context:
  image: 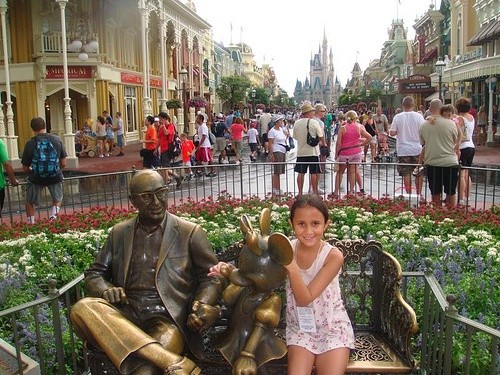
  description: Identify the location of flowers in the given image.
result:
[186,95,208,108]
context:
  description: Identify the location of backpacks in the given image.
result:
[31,133,61,179]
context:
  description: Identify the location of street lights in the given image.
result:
[435,57,446,103]
[179,65,189,134]
[383,81,390,119]
[251,89,256,114]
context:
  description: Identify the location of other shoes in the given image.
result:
[105,153,110,157]
[175,175,184,190]
[116,152,123,156]
[222,160,230,164]
[328,192,339,198]
[184,171,216,182]
[236,161,241,165]
[360,189,367,195]
[167,177,172,184]
[250,155,256,163]
[344,194,356,199]
[98,154,104,157]
[413,166,425,176]
[314,189,326,195]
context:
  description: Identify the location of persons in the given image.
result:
[328,110,372,200]
[73,170,228,375]
[413,98,476,209]
[390,96,427,205]
[468,104,497,146]
[293,102,331,196]
[75,111,125,158]
[137,106,246,187]
[0,139,19,226]
[247,100,394,172]
[268,114,290,196]
[20,118,67,225]
[210,195,357,375]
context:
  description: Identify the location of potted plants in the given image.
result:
[166,99,182,109]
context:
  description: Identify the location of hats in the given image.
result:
[301,104,317,113]
[272,114,283,123]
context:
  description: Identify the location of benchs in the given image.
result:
[82,237,420,375]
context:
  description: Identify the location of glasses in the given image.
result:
[321,110,327,113]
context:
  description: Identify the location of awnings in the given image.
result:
[441,54,500,82]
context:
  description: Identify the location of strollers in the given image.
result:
[218,136,242,164]
[377,133,393,163]
[75,133,97,157]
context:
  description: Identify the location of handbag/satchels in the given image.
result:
[458,116,471,142]
[286,145,291,151]
[289,137,295,149]
[209,132,217,144]
[307,132,319,147]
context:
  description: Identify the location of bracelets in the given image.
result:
[269,155,274,159]
